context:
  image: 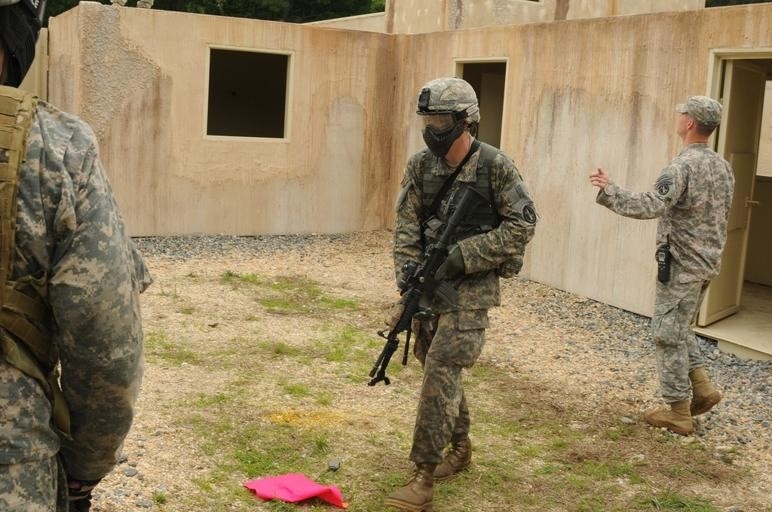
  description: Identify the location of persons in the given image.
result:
[381,75,544,511]
[1,0,156,512]
[588,95,736,436]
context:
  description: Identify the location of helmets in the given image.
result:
[417,77,478,111]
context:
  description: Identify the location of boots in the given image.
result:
[645,399,694,437]
[688,366,723,417]
[383,463,438,512]
[432,430,472,481]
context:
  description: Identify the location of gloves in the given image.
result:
[57,476,105,512]
[432,244,465,282]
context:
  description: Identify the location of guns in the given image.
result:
[367,186,489,386]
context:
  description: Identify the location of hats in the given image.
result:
[675,95,725,127]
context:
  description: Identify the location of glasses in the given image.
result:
[416,102,480,134]
